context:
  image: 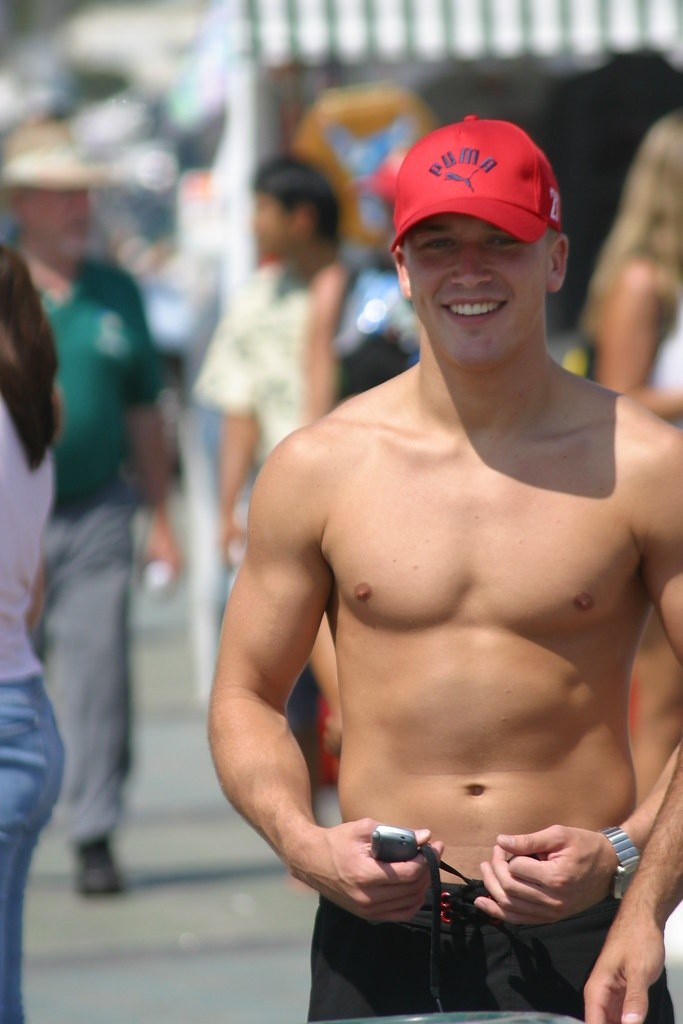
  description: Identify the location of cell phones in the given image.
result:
[369,825,418,864]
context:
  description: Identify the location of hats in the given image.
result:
[0,118,112,193]
[390,114,561,252]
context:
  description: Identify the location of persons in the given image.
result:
[210,117,683,1024]
[2,119,186,896]
[1,39,683,831]
[583,744,683,1024]
[1,245,66,1023]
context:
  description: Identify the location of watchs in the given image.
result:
[599,826,641,900]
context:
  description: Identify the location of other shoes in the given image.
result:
[73,842,121,895]
